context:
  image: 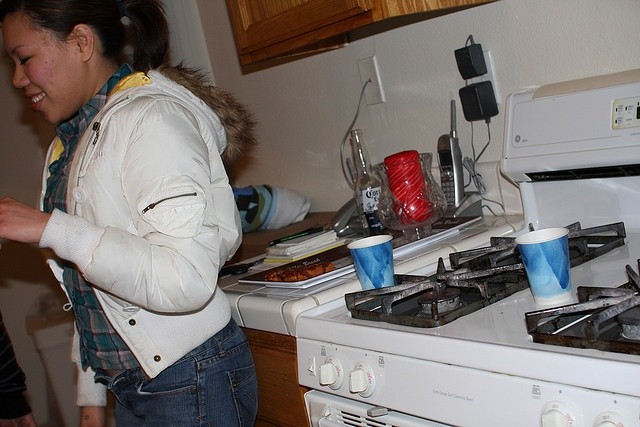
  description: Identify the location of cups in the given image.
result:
[346,235,395,291]
[371,149,449,243]
[515,229,572,307]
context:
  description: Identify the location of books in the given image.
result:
[248,226,347,272]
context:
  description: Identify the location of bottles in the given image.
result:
[350,128,387,237]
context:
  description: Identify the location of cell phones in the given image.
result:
[437,100,465,209]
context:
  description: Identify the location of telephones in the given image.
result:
[437,99,483,218]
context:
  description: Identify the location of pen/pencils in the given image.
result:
[268,227,323,246]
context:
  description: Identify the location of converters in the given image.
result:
[454,34,488,80]
[458,81,499,122]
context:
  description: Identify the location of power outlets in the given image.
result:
[466,49,501,104]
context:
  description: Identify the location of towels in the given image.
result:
[231,184,310,232]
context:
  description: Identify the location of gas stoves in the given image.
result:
[296,220,640,427]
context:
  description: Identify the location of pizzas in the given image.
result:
[263,260,336,282]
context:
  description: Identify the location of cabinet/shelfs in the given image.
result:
[225,0,500,76]
[239,325,310,427]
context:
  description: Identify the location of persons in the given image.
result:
[0,308,37,427]
[0,0,260,427]
